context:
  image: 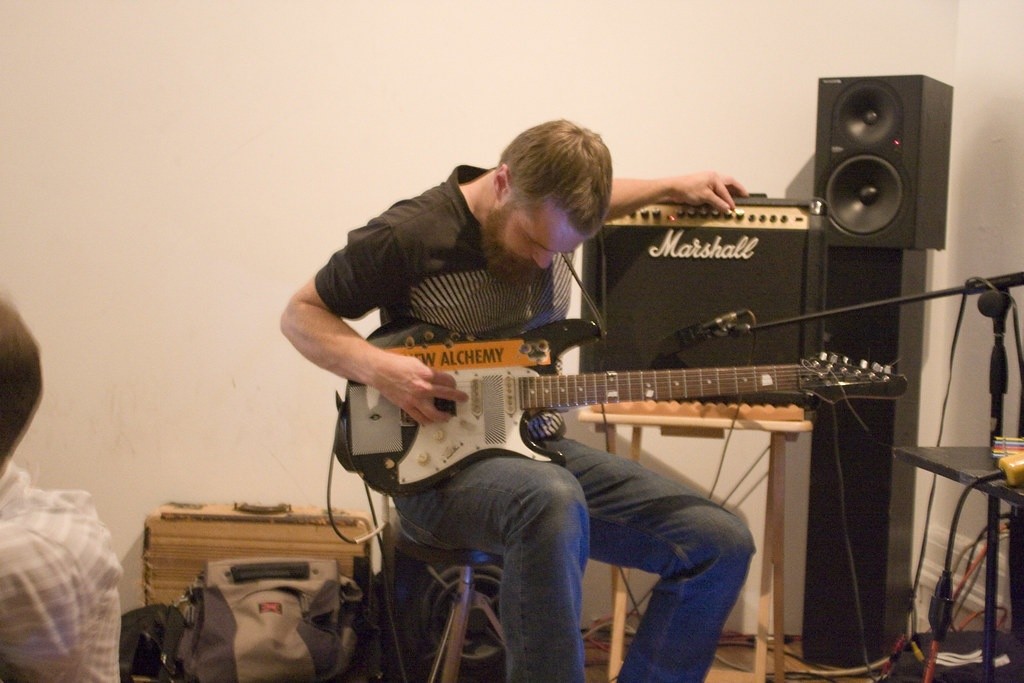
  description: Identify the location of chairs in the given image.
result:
[378,492,503,683]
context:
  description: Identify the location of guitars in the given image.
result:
[341,318,911,496]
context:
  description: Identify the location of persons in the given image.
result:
[279,121,755,683]
[0,303,122,683]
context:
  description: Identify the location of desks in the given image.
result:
[894,446,1024,683]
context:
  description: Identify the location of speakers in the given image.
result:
[814,74,955,252]
[390,534,509,683]
[579,196,828,413]
[802,248,927,672]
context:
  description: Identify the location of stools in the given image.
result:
[578,403,813,683]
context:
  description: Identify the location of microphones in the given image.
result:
[675,310,751,348]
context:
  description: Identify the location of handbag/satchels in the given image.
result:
[118,603,187,683]
[176,557,372,683]
